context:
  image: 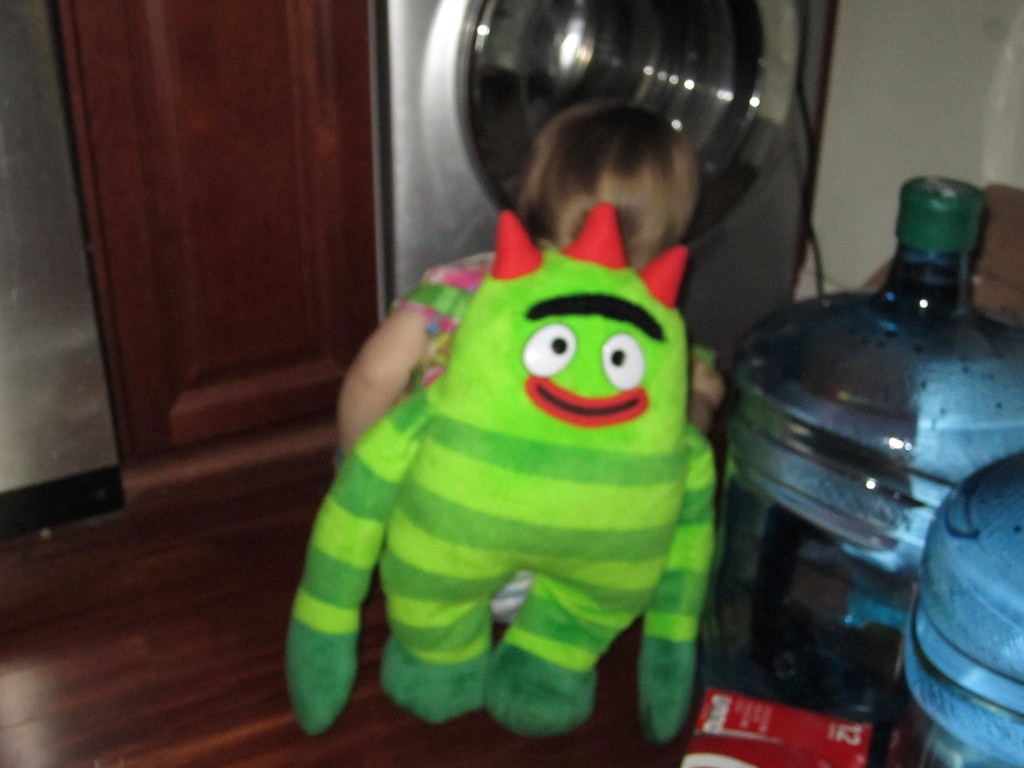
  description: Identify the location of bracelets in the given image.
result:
[336,453,343,466]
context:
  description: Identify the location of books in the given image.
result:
[679,687,871,768]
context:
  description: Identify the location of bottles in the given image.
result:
[870,450,1023,764]
[693,170,1024,727]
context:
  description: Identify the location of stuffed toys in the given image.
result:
[285,202,716,745]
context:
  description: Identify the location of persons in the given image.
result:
[332,101,726,621]
